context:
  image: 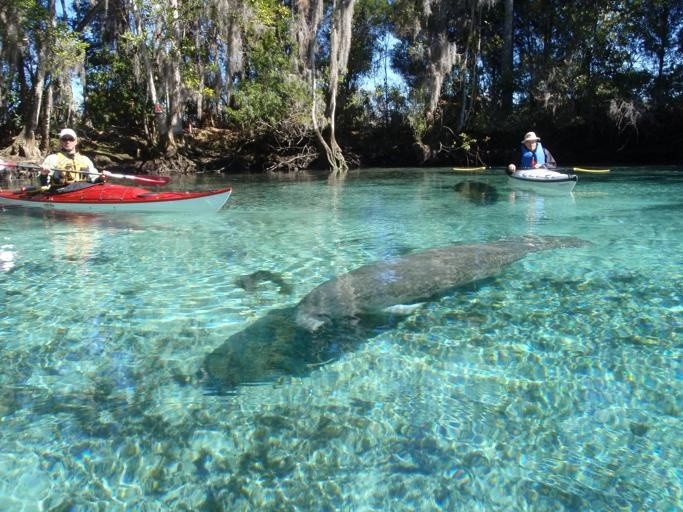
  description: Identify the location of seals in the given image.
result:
[294,236,596,351]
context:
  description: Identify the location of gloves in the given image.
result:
[508,163,515,172]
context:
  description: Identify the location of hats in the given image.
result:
[59,128,76,139]
[521,131,540,142]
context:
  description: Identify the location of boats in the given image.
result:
[511,169,578,181]
[0,181,233,213]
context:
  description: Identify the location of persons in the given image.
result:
[507,131,557,174]
[38,127,111,195]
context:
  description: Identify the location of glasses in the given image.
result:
[61,137,74,141]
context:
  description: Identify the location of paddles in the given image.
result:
[453,167,610,173]
[0,160,169,186]
